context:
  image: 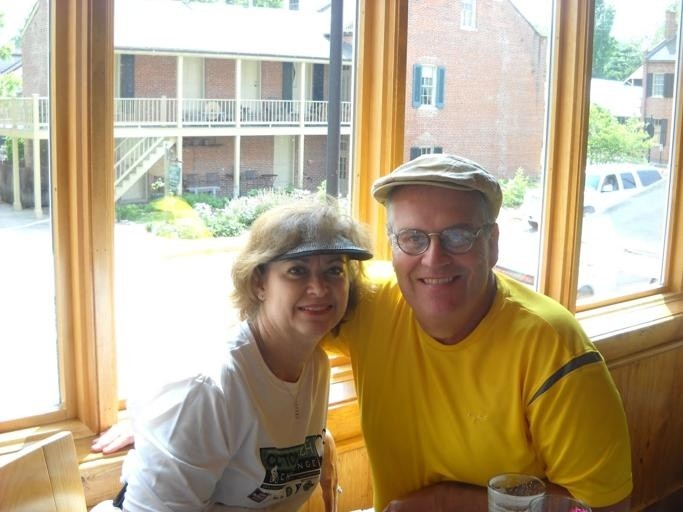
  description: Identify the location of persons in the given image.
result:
[88,198,376,512]
[88,153,637,512]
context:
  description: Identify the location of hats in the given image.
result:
[370,155,502,218]
[278,212,372,261]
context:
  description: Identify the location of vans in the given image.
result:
[522,164,664,230]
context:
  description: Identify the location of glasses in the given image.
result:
[389,225,488,257]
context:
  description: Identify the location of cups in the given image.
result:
[486,472,593,512]
